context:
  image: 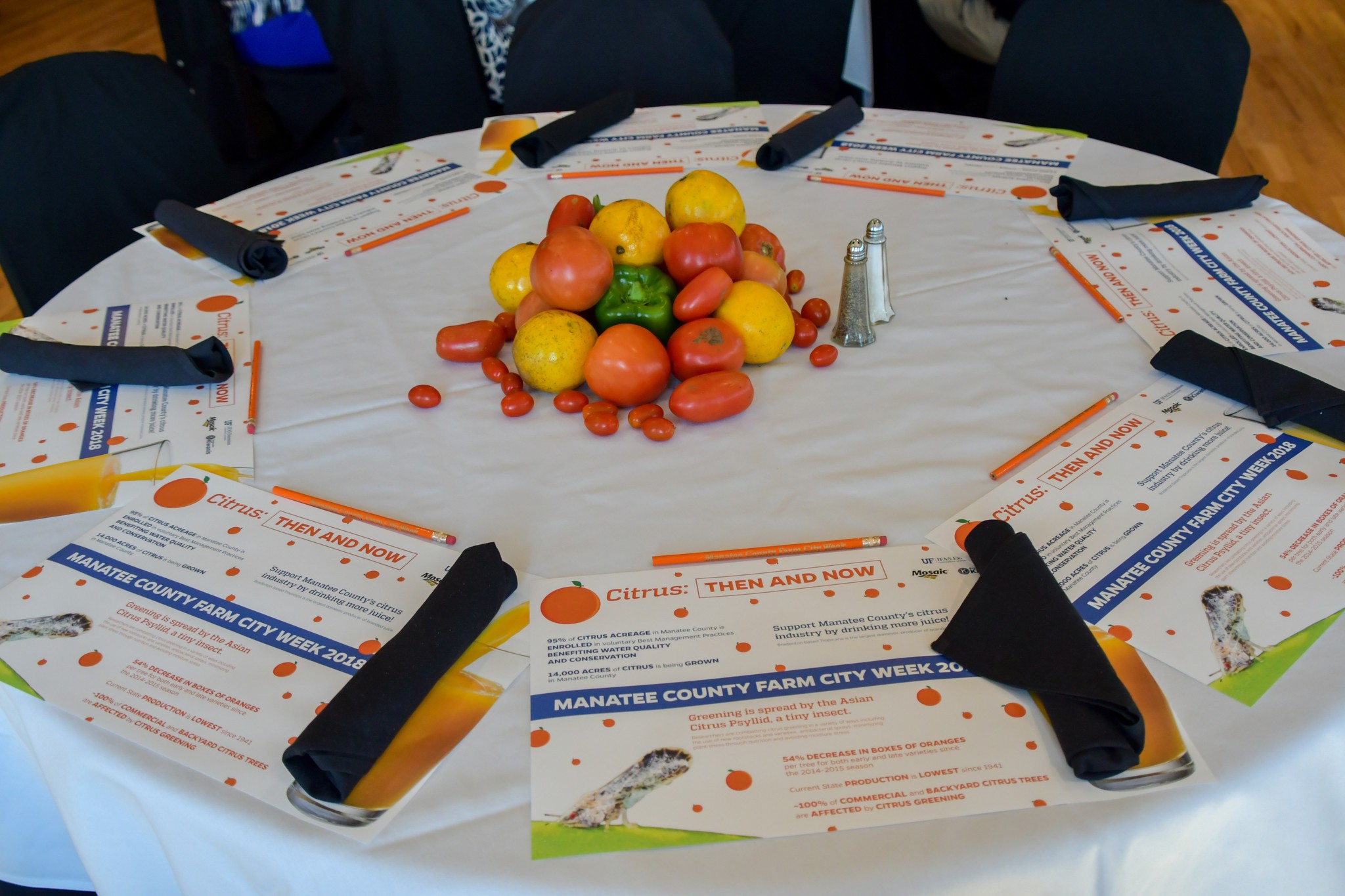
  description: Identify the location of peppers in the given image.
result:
[593,263,679,345]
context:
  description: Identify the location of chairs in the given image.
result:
[0,47,232,334]
[988,0,1256,180]
[500,0,740,121]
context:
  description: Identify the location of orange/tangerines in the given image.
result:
[713,280,796,365]
[489,241,539,312]
[665,169,746,238]
[588,198,672,268]
[512,309,598,393]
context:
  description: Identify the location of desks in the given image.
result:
[0,102,1344,896]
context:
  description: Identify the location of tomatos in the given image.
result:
[435,320,507,363]
[584,323,670,409]
[669,371,754,423]
[782,292,838,367]
[513,291,552,331]
[667,317,746,381]
[662,222,743,286]
[786,269,805,294]
[739,223,786,272]
[673,267,734,323]
[553,389,619,436]
[546,194,595,236]
[408,384,441,409]
[628,403,676,442]
[482,357,534,418]
[529,226,614,314]
[494,311,517,341]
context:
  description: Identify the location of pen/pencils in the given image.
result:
[345,206,471,257]
[1049,246,1124,323]
[547,166,684,180]
[272,484,458,546]
[991,392,1118,481]
[247,340,261,435]
[651,536,888,568]
[806,175,945,198]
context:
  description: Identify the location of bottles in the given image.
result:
[863,217,894,323]
[830,238,877,347]
